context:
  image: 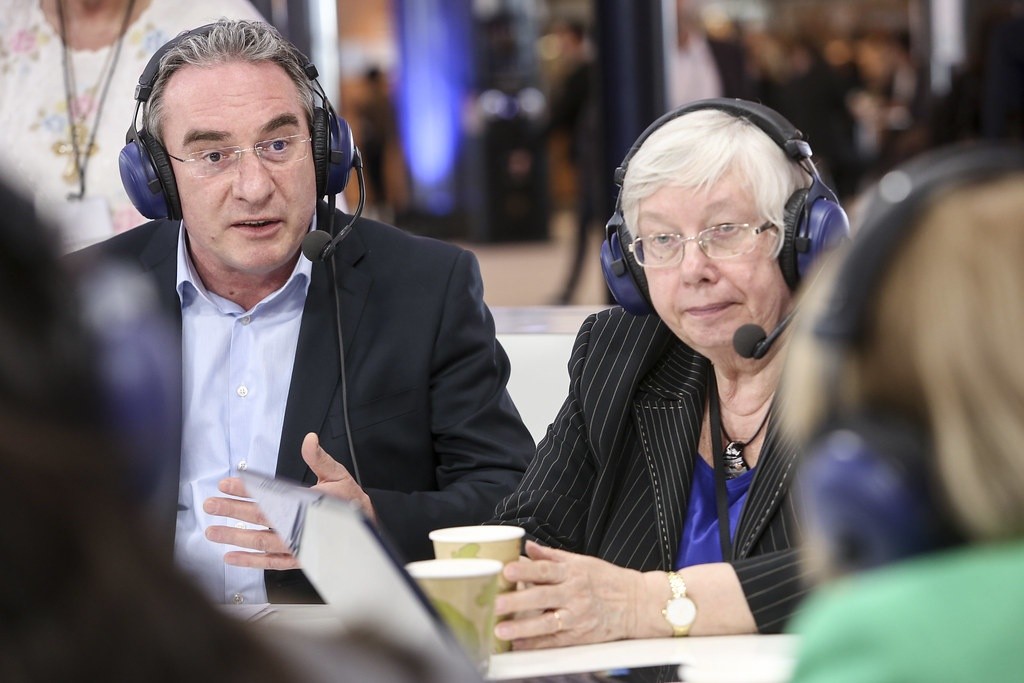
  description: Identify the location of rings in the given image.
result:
[551,610,565,632]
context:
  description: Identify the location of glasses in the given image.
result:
[628,221,775,268]
[170,133,313,177]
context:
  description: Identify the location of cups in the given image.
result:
[428,525,526,656]
[403,557,504,677]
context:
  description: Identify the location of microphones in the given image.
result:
[302,147,366,263]
[733,236,851,359]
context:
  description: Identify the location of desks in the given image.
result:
[217,603,793,683]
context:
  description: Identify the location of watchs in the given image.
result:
[662,569,697,637]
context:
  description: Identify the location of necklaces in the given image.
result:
[52,0,133,200]
[720,392,777,476]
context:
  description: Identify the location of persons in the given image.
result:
[0,0,1024,683]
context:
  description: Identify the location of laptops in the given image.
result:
[236,467,690,683]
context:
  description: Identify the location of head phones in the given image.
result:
[602,97,850,317]
[119,21,354,222]
[800,142,1024,567]
